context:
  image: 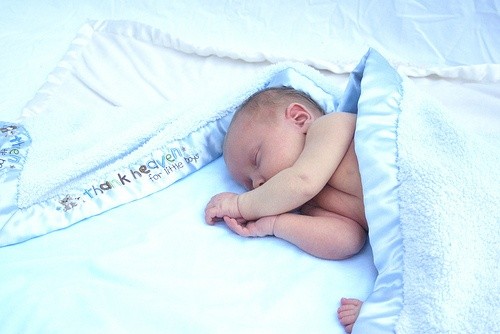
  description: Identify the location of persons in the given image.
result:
[204,85,370,334]
